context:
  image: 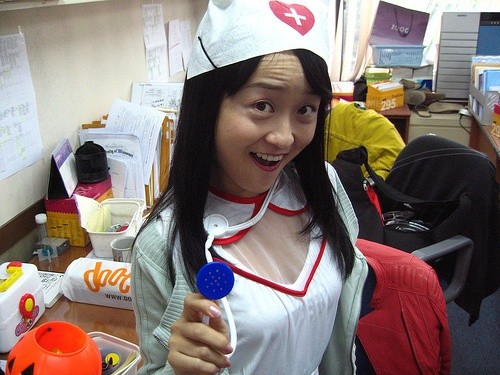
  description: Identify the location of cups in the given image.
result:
[491,104,500,137]
[111,236,137,263]
[365,67,392,87]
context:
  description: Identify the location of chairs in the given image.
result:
[356,241,451,375]
[338,135,499,326]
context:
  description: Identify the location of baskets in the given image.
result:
[370,44,427,66]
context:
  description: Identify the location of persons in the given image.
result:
[130,0,370,375]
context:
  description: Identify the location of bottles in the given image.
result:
[33,213,62,273]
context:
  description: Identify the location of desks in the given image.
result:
[0,242,148,375]
[468,109,499,163]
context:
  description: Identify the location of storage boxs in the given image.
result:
[86,199,140,258]
[365,45,427,111]
[86,332,142,375]
[468,83,491,126]
[0,261,46,354]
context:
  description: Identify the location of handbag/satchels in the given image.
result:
[331,146,472,280]
[369,0,429,44]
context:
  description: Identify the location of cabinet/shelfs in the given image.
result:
[364,105,410,146]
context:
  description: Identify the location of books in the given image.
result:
[470,56,500,122]
[37,270,67,308]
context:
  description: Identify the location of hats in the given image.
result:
[186,0,330,79]
